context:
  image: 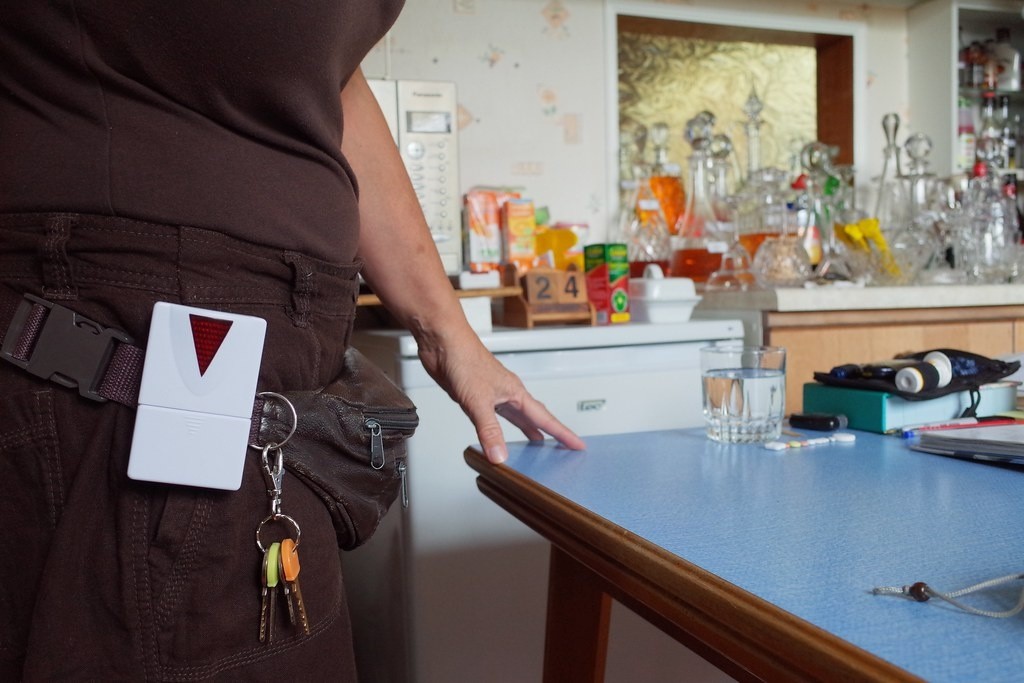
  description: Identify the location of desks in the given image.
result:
[464,415,1024,683]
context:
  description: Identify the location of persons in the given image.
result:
[0,0,581,683]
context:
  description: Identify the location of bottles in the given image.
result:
[620,96,872,285]
[957,95,1023,168]
[959,24,1022,91]
[875,113,1023,283]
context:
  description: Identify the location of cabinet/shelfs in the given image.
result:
[764,303,1024,412]
[908,2,1024,195]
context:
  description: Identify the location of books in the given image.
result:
[910,424,1024,463]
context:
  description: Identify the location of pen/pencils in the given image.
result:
[903,419,1024,438]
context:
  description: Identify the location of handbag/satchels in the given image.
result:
[257,345,419,551]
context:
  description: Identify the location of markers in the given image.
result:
[901,414,1015,433]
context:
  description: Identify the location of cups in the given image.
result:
[753,237,832,286]
[699,345,785,442]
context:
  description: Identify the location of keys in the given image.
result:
[260,539,309,646]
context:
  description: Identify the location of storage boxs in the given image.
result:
[585,242,631,325]
